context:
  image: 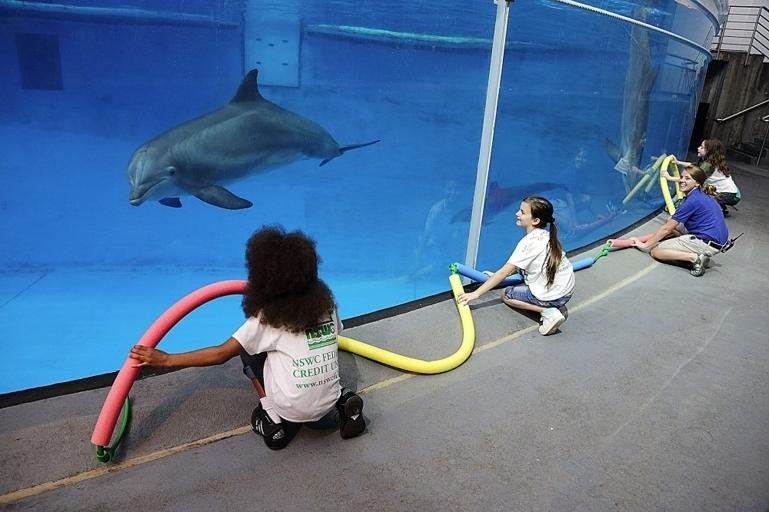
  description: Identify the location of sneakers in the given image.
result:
[690,253,710,276]
[335,387,365,439]
[251,402,288,450]
[539,308,565,336]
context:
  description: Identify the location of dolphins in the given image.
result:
[606,5,661,203]
[128,69,381,211]
[449,182,573,226]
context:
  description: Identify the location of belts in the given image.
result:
[704,240,721,250]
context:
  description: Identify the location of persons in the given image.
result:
[661,137,741,216]
[411,179,462,279]
[457,196,576,336]
[128,222,365,451]
[558,145,605,227]
[607,129,655,193]
[629,165,728,277]
